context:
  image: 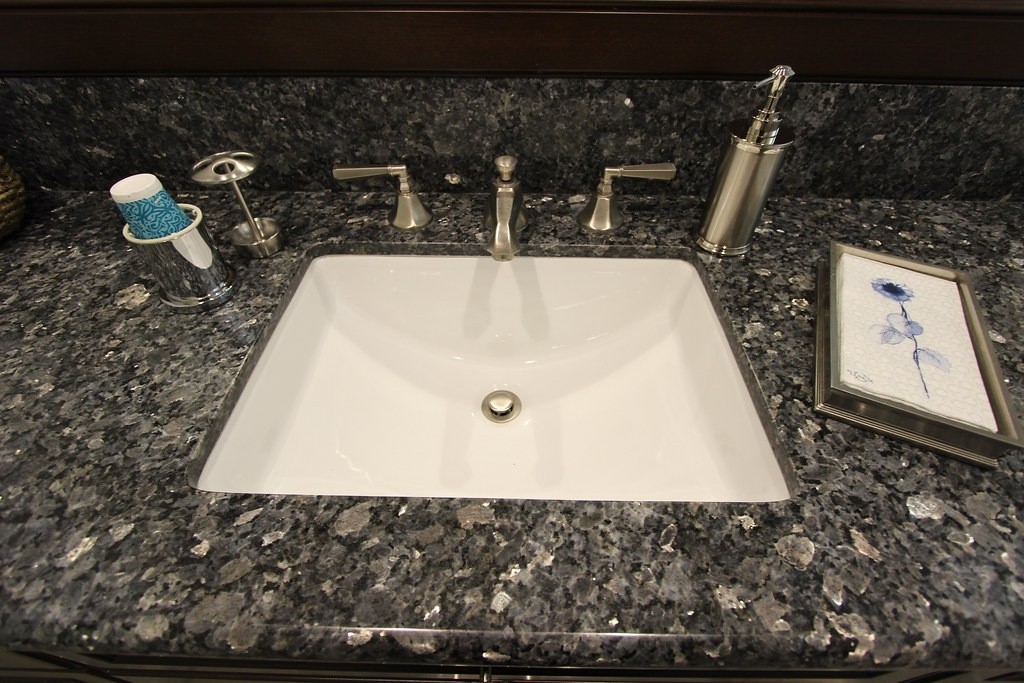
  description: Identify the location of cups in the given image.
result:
[109,173,194,240]
[120,203,235,304]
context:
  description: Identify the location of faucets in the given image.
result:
[485,156,531,261]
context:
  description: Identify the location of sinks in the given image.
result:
[194,255,790,502]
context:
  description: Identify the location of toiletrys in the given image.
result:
[693,65,796,256]
